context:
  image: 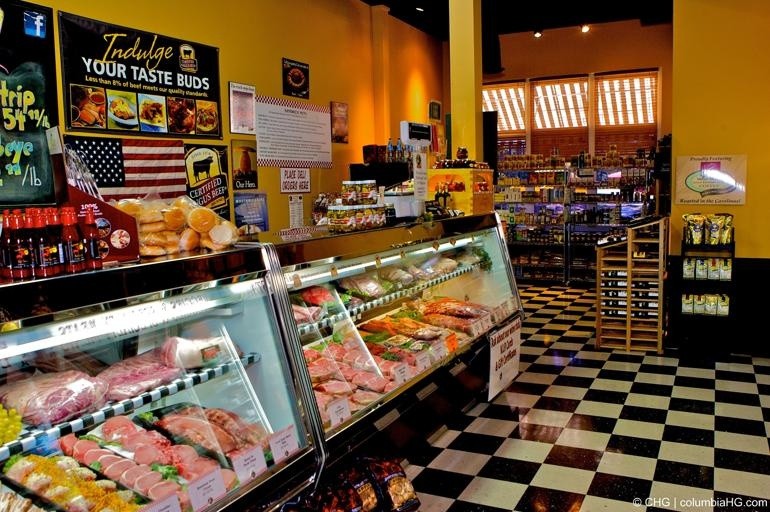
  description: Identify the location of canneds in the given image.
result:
[327,179,396,233]
[585,143,648,168]
[435,146,490,169]
[497,147,565,171]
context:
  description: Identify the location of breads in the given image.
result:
[117,198,240,257]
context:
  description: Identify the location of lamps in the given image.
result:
[581,24,589,33]
[534,30,543,38]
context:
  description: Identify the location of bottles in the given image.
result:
[0,207,102,279]
[387,138,412,163]
[599,228,659,317]
[435,182,451,209]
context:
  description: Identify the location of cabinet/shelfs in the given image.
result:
[497,166,656,287]
[595,217,735,356]
[0,213,526,512]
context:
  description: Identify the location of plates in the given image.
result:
[140,99,165,127]
[107,94,140,126]
